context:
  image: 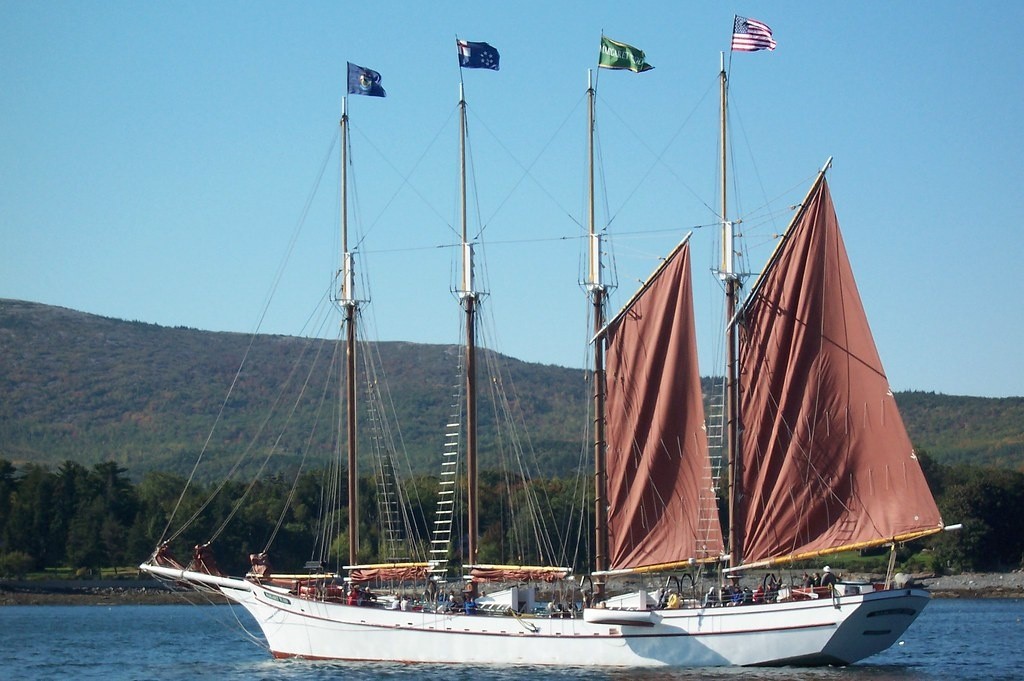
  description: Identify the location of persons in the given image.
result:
[347,583,607,619]
[655,588,680,609]
[702,565,836,610]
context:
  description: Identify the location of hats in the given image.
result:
[823,566,831,572]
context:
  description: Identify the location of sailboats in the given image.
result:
[139,51,962,666]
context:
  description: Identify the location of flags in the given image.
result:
[598,33,655,73]
[456,39,501,72]
[731,14,776,52]
[346,62,387,98]
